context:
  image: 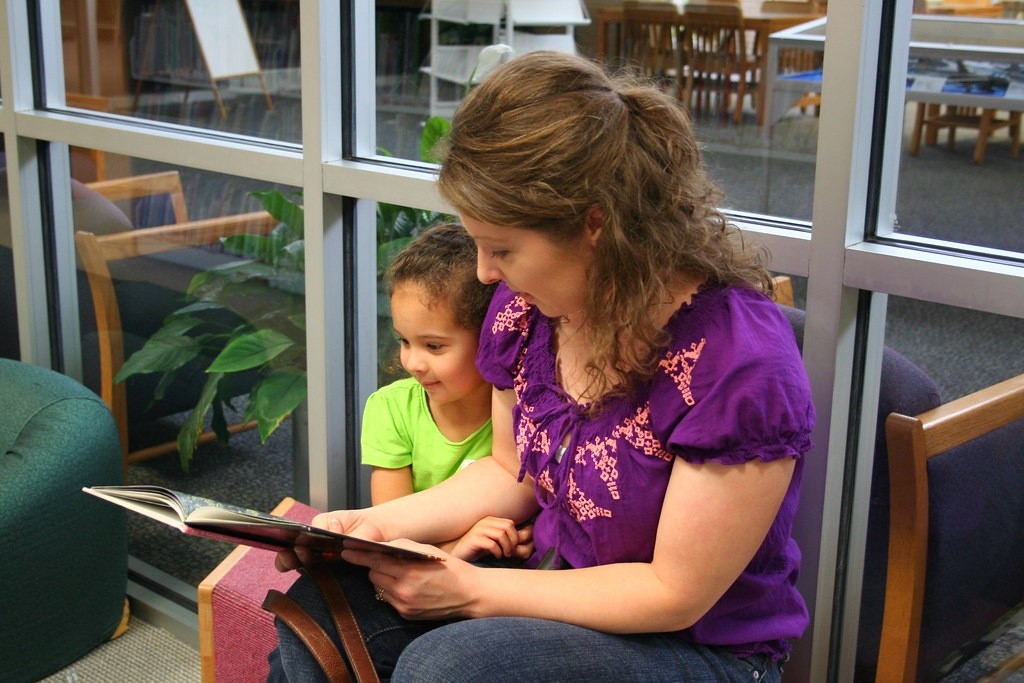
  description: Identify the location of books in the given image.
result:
[82,485,446,570]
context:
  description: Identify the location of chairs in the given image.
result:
[592,0,1024,163]
[874,372,1023,683]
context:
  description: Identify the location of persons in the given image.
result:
[259,48,813,682]
[262,220,536,681]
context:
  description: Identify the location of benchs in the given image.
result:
[198,493,323,681]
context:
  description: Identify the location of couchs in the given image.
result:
[754,269,939,683]
[2,168,305,486]
[0,357,131,683]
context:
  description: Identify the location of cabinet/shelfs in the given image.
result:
[416,1,593,130]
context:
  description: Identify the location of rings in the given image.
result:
[376,589,384,602]
